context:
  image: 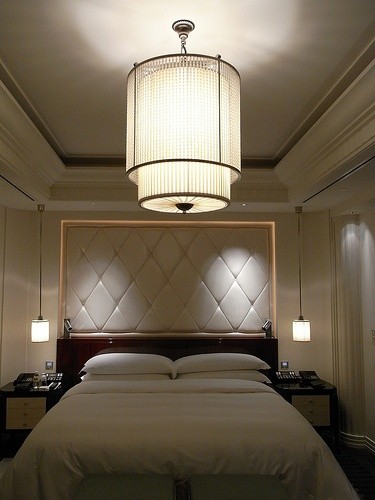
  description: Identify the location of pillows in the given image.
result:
[78,353,177,380]
[174,353,272,384]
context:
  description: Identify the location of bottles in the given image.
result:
[41,373,47,386]
[33,371,39,389]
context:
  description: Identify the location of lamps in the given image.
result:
[126,20,242,214]
[31,204,49,342]
[292,206,310,342]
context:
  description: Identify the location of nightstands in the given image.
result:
[0,382,61,458]
[282,380,338,450]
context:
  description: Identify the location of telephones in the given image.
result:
[13,372,35,385]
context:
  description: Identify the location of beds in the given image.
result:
[0,337,360,500]
